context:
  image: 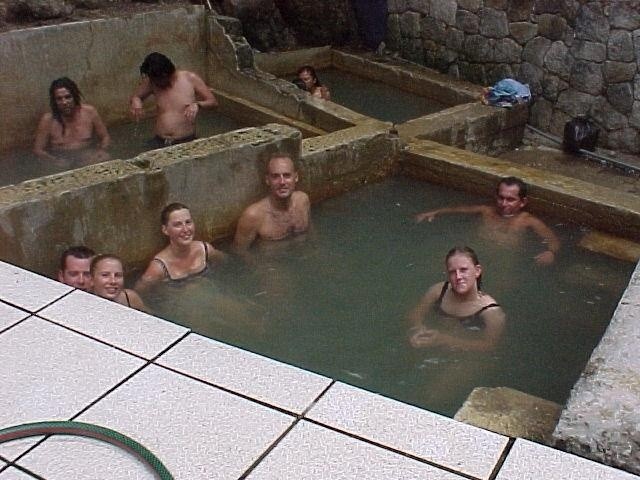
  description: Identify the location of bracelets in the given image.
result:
[548,249,560,258]
[192,100,201,110]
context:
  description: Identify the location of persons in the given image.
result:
[132,203,261,344]
[58,244,95,293]
[407,245,505,357]
[89,254,150,314]
[298,65,331,102]
[416,175,563,278]
[232,155,313,268]
[129,52,219,149]
[30,76,111,169]
